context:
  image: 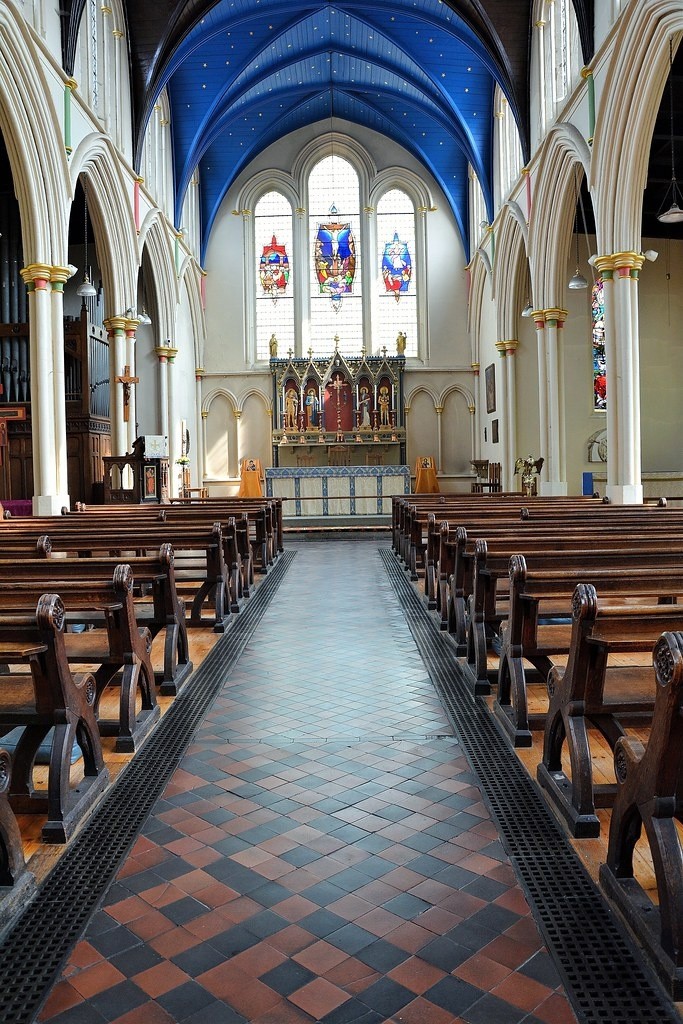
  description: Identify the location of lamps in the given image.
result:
[567,166,590,292]
[75,175,97,299]
[521,264,534,319]
[329,53,339,217]
[140,256,152,325]
[656,79,683,224]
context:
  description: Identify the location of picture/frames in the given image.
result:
[491,419,499,443]
[141,463,161,502]
[484,363,497,414]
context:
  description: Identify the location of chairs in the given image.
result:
[365,451,385,466]
[326,446,352,466]
[295,454,313,467]
[184,469,208,504]
[470,461,502,492]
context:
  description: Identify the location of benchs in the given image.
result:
[390,491,683,1001]
[0,496,285,941]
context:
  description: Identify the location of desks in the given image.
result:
[263,465,412,517]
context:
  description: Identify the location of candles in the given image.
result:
[300,386,304,413]
[355,384,360,412]
[391,384,395,409]
[374,384,378,411]
[282,386,286,412]
[318,385,322,412]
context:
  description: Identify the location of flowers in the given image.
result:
[177,456,191,468]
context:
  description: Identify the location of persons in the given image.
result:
[285,391,298,427]
[269,334,278,357]
[378,388,392,427]
[247,460,256,471]
[396,331,407,355]
[421,458,429,468]
[359,388,371,426]
[305,390,319,427]
